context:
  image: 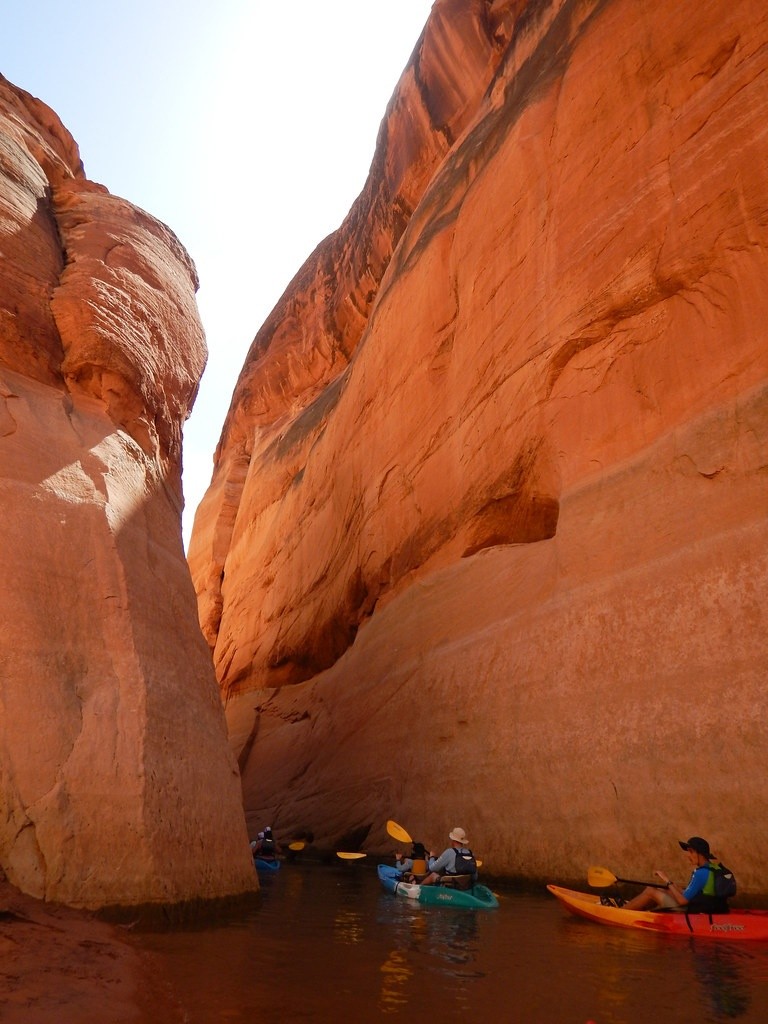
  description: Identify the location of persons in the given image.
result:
[599,836,733,914]
[252,827,280,855]
[419,827,478,889]
[396,844,431,885]
[250,832,264,849]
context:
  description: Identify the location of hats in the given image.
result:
[678,838,718,859]
[257,827,271,839]
[448,828,469,844]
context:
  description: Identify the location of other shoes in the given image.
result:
[599,893,626,908]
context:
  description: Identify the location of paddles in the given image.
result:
[588,866,685,891]
[280,842,305,850]
[337,820,482,867]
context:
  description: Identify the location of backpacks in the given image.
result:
[713,863,736,898]
[451,847,476,876]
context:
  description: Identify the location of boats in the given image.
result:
[546,883,767,943]
[378,865,500,908]
[254,859,280,879]
